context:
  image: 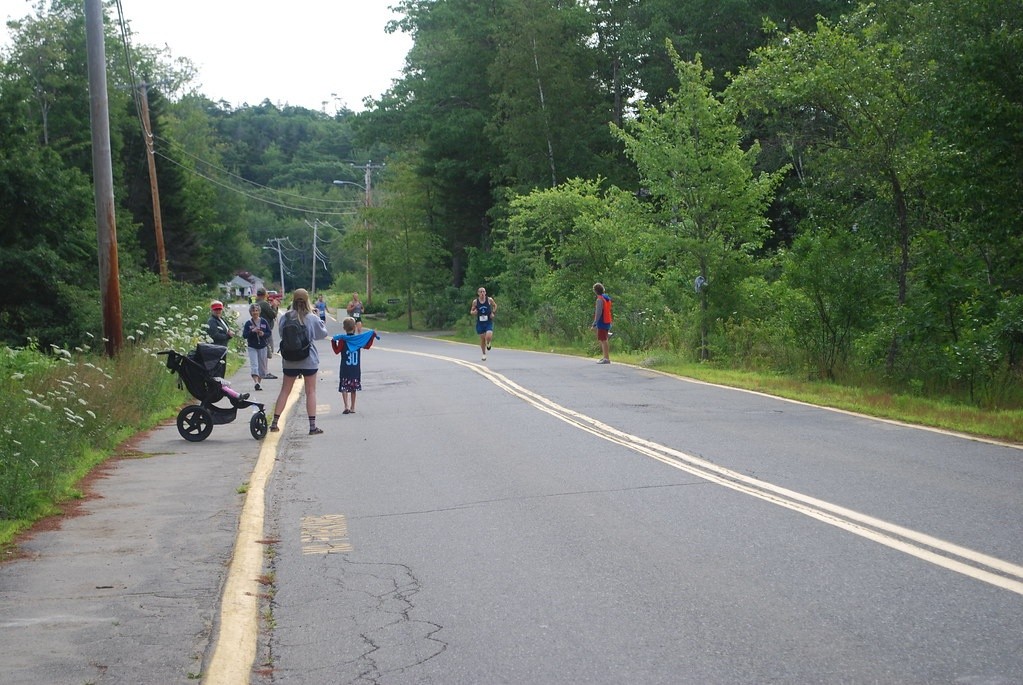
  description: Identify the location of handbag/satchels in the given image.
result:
[267,346,272,358]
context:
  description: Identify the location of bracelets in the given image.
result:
[493,312,495,315]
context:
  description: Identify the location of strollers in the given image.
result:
[156,342,270,443]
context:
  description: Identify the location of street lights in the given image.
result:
[262,241,284,302]
[333,170,372,307]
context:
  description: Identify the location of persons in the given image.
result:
[243,288,293,390]
[331,317,377,414]
[591,283,612,364]
[270,288,328,435]
[212,377,250,401]
[312,293,329,325]
[205,301,235,379]
[471,287,497,361]
[347,292,364,334]
[219,291,232,307]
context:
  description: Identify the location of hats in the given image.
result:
[294,288,308,301]
[211,303,223,310]
[257,288,266,294]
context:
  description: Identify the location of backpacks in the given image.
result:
[279,312,315,361]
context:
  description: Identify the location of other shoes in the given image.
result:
[343,409,356,414]
[596,357,610,364]
[270,426,279,431]
[255,383,260,391]
[482,355,486,360]
[238,393,249,402]
[264,373,277,379]
[310,427,323,434]
[486,343,491,350]
